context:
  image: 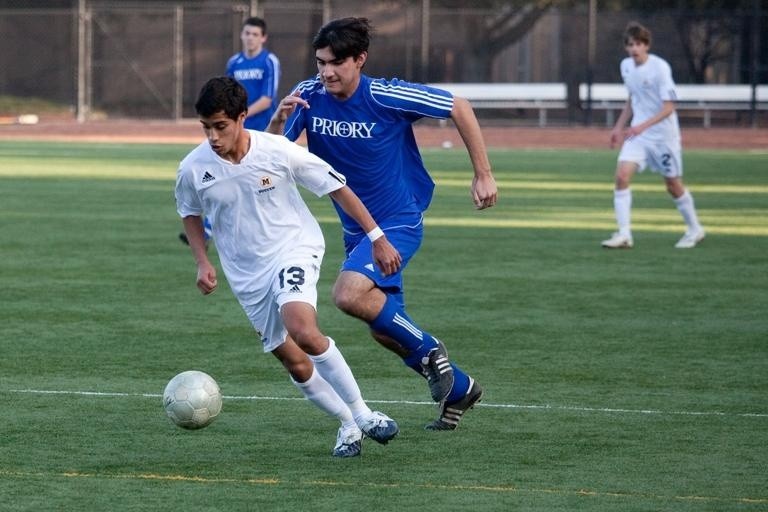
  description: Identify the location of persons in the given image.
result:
[179,21,282,253]
[600,21,704,249]
[265,18,498,430]
[176,77,404,458]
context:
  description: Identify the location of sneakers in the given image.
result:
[360,411,401,446]
[423,375,484,431]
[417,335,455,409]
[333,425,368,457]
[601,232,635,249]
[674,226,705,249]
[179,232,209,251]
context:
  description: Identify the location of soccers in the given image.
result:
[443,141,453,149]
[162,371,222,428]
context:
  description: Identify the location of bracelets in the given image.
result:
[367,228,383,242]
